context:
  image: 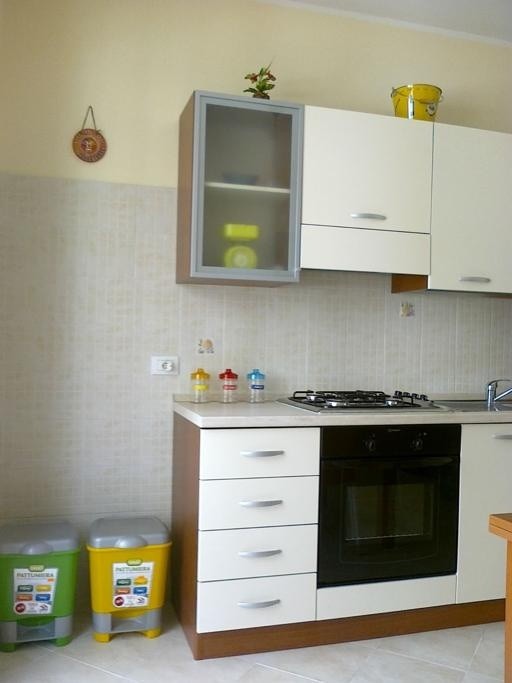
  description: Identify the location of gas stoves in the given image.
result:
[277,388,449,411]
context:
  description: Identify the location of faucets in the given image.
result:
[485,379,512,405]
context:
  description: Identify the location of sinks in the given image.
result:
[430,399,499,413]
[493,401,512,413]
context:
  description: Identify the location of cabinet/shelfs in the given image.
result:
[192,426,321,636]
[174,87,305,289]
[456,424,512,606]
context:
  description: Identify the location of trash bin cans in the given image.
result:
[0,522,83,653]
[85,515,174,642]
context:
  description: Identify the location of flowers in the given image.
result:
[242,61,277,96]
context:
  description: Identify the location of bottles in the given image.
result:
[218,368,239,403]
[190,367,211,402]
[246,368,265,402]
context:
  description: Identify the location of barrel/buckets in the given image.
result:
[389,84,443,121]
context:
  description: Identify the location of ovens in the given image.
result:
[315,425,460,591]
